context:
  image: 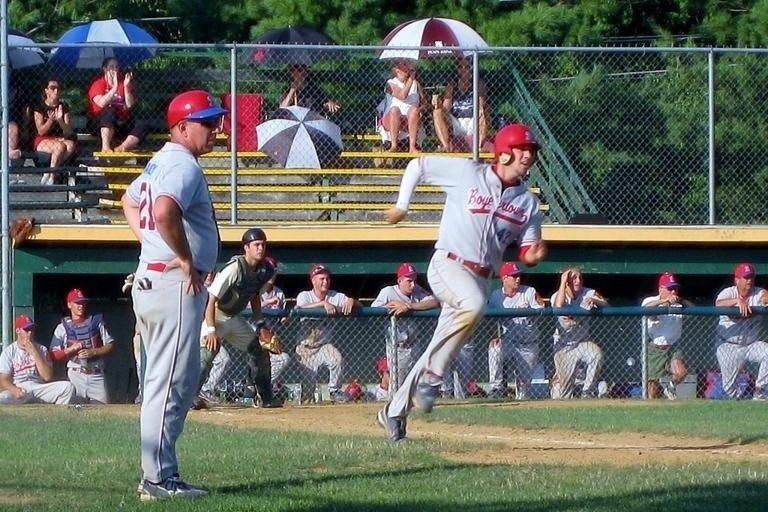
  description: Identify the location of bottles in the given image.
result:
[234,398,253,404]
[288,383,302,406]
[313,379,322,403]
[220,387,226,401]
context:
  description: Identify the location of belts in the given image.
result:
[72,367,102,374]
[448,253,492,278]
[148,262,167,271]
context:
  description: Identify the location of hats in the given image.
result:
[15,315,38,330]
[310,263,328,277]
[736,263,755,278]
[659,272,679,288]
[500,262,522,277]
[397,263,417,277]
[377,360,390,372]
[67,289,89,303]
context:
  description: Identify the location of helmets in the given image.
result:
[242,228,266,245]
[494,124,542,164]
[167,90,228,130]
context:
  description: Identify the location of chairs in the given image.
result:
[569,213,609,225]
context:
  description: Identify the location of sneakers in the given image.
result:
[664,384,677,400]
[754,390,767,401]
[138,477,209,501]
[377,402,410,446]
[198,390,219,404]
[40,173,59,184]
[264,397,281,408]
[415,382,442,413]
[330,389,349,403]
[485,388,511,398]
[581,389,595,399]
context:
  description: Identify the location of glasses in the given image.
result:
[456,64,472,70]
[49,85,61,92]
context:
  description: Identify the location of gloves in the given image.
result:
[136,278,152,290]
[122,272,134,293]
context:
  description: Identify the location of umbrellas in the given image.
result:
[47,18,160,96]
[380,15,494,107]
[256,108,345,170]
[3,31,45,86]
[237,23,340,104]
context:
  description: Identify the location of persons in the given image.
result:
[123,229,441,411]
[7,83,23,160]
[438,262,696,400]
[276,62,341,118]
[86,57,148,153]
[716,264,768,401]
[119,88,232,502]
[47,289,116,404]
[29,78,77,188]
[0,315,75,406]
[376,123,549,440]
[377,59,425,155]
[430,56,488,153]
